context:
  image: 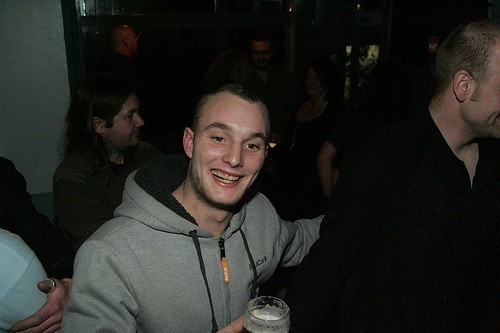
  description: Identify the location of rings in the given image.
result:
[46,278,55,292]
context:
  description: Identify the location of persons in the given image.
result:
[287,19,500,333]
[1,157,76,333]
[59,79,324,333]
[234,29,305,175]
[271,50,355,299]
[0,228,51,333]
[52,76,171,281]
[102,23,153,141]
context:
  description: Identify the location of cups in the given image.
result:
[243,295,290,332]
